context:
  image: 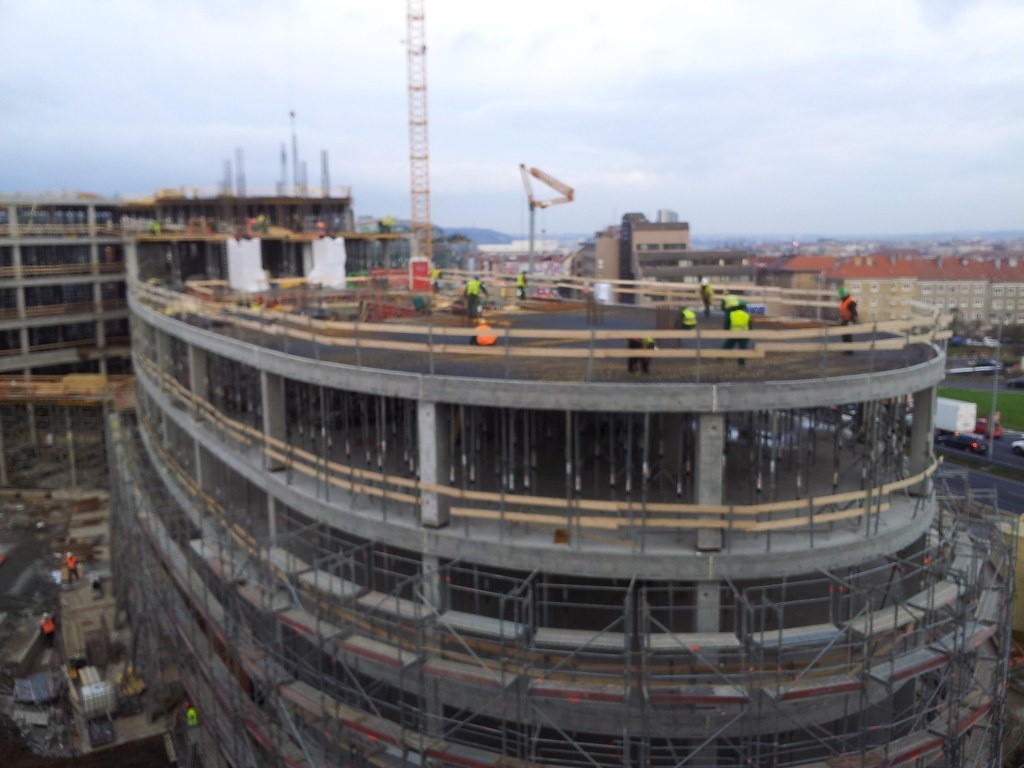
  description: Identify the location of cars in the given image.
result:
[943,433,989,455]
[951,336,1002,349]
[977,418,1003,439]
[1011,438,1024,455]
[768,403,857,440]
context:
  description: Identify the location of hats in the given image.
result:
[701,277,709,286]
[67,552,72,558]
[43,612,49,618]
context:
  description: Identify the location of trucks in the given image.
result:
[905,396,977,444]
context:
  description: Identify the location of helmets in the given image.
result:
[839,288,848,297]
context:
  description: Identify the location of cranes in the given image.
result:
[407,1,433,259]
[521,162,575,273]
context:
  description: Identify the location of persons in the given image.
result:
[697,274,714,317]
[837,288,859,356]
[674,305,698,345]
[430,267,443,294]
[464,276,490,318]
[65,552,80,585]
[40,612,56,648]
[718,292,753,367]
[147,214,396,237]
[183,701,199,728]
[516,267,529,300]
[995,411,1000,424]
[470,318,497,345]
[627,338,656,374]
